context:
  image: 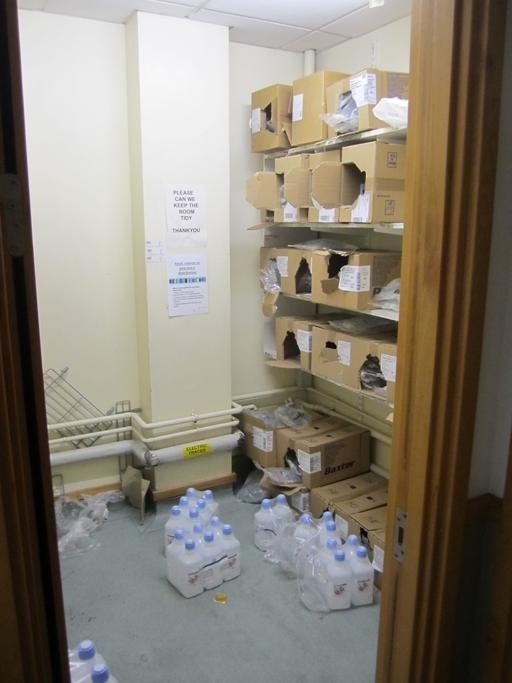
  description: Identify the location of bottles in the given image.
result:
[293,511,374,611]
[254,495,293,553]
[76,640,117,683]
[164,487,241,599]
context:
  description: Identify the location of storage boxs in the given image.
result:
[244,69,406,592]
